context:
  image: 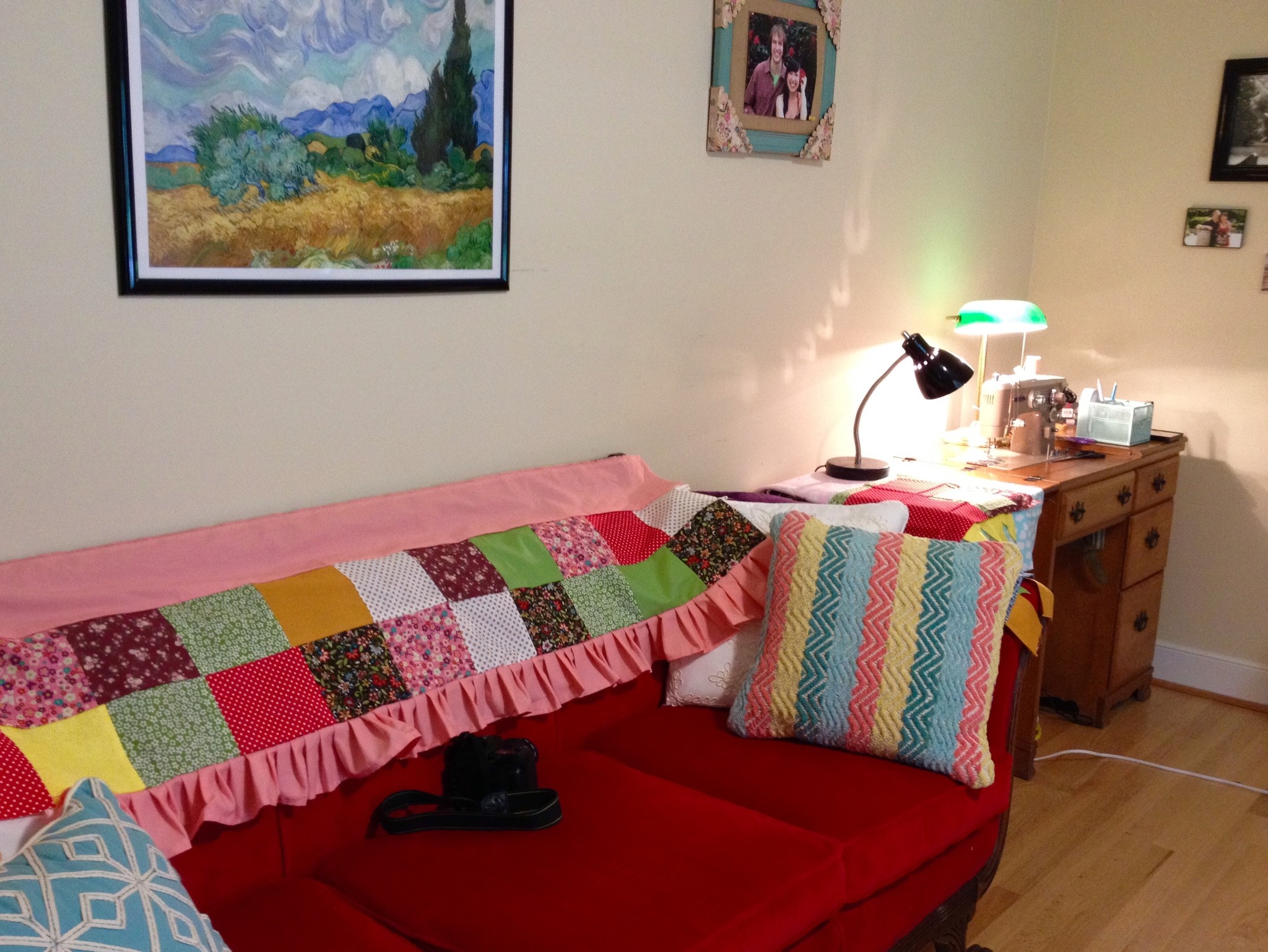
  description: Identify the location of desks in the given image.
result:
[769,422,1185,785]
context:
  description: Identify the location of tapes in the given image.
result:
[1055,422,1067,430]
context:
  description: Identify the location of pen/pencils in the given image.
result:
[1111,380,1117,400]
[1096,378,1105,402]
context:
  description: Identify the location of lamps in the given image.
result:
[810,332,974,484]
[945,295,1053,465]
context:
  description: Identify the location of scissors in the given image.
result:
[1051,450,1106,462]
[1054,436,1096,445]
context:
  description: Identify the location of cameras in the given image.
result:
[441,731,538,794]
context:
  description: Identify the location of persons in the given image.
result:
[744,24,788,116]
[776,61,808,120]
[1196,210,1221,246]
[1217,212,1231,247]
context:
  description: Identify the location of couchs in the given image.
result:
[4,453,1053,949]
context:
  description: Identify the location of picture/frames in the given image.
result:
[1183,208,1248,248]
[703,0,841,164]
[99,0,514,297]
[1209,59,1268,187]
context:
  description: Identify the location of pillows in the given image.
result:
[728,500,1026,793]
[5,780,237,952]
[656,487,915,714]
